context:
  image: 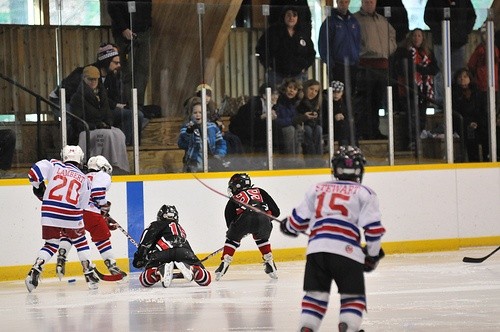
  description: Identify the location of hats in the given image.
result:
[330,80,344,92]
[83,66,100,79]
[97,42,120,61]
[195,84,211,97]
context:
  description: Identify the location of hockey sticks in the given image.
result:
[463,247,500,264]
[107,215,139,250]
[172,246,224,279]
[92,267,122,281]
[193,173,311,236]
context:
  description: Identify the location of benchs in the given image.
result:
[0,101,499,179]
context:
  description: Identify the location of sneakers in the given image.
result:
[215,261,230,281]
[263,253,279,279]
[155,261,195,288]
[24,247,128,294]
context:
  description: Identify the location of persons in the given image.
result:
[227,79,356,154]
[131,204,211,290]
[54,153,127,281]
[398,27,441,148]
[68,66,130,173]
[269,0,313,45]
[423,0,477,106]
[376,0,410,48]
[452,68,490,162]
[177,97,233,172]
[25,146,100,292]
[195,85,244,154]
[468,24,500,161]
[254,5,316,90]
[350,0,398,140]
[317,0,364,139]
[46,42,144,146]
[278,143,386,332]
[106,0,151,115]
[215,173,281,280]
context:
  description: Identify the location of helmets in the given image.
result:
[87,155,113,173]
[228,173,252,198]
[331,144,368,183]
[60,144,84,169]
[157,204,179,223]
